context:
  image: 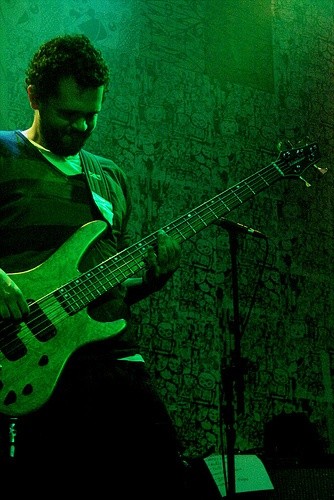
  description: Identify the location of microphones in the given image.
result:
[214,216,267,239]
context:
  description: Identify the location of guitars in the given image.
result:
[0,136,329,418]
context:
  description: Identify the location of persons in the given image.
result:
[0,33,197,500]
